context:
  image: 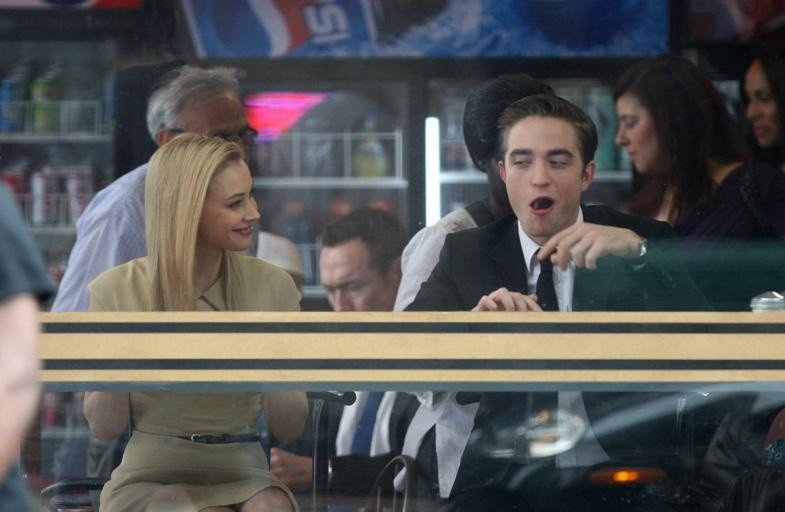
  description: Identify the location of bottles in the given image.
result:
[251,106,389,286]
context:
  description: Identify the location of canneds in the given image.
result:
[0,58,103,285]
[41,389,85,428]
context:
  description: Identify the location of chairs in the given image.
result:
[303,389,486,511]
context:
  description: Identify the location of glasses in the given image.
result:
[162,126,262,150]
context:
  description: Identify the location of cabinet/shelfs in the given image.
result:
[0,28,632,300]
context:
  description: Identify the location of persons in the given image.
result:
[265,203,442,498]
[1,177,60,511]
[79,132,311,510]
[48,63,261,481]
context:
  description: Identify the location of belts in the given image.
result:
[173,432,263,444]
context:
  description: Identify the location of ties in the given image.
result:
[526,257,562,474]
[349,391,388,458]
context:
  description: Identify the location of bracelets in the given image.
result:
[327,458,333,493]
